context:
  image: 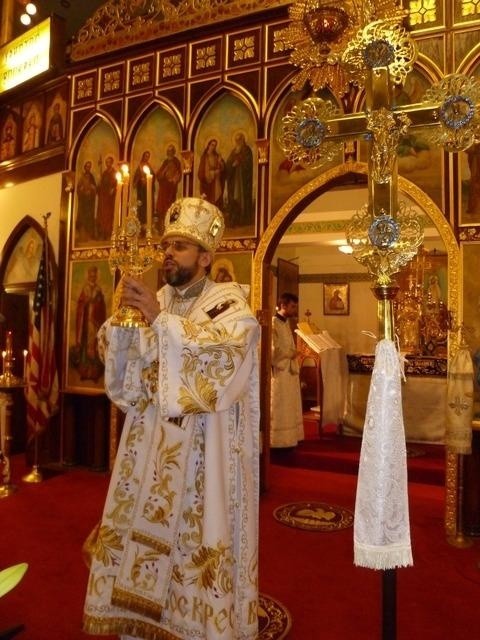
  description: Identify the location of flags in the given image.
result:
[21,222,60,444]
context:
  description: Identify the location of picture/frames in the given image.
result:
[323,282,350,316]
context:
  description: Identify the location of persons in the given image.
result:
[24,240,37,260]
[75,267,107,388]
[154,143,184,231]
[75,160,96,244]
[80,195,261,640]
[270,293,308,449]
[198,139,226,211]
[429,275,443,302]
[211,260,235,286]
[96,157,116,240]
[0,121,18,161]
[131,149,157,236]
[22,111,41,151]
[223,132,254,227]
[44,102,65,145]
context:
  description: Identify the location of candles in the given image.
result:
[112,163,154,229]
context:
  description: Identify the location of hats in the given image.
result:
[160,197,225,254]
